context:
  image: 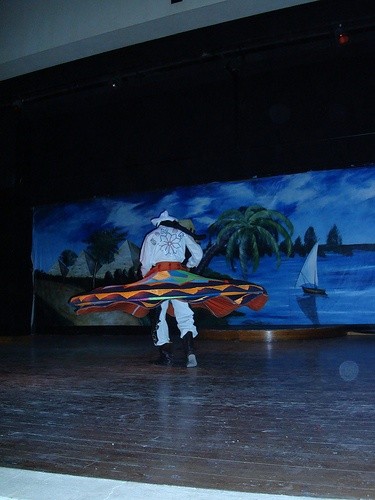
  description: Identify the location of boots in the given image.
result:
[156,344,176,365]
[183,332,197,367]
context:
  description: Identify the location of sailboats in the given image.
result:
[296,241,326,295]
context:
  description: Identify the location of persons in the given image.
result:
[141,211,204,368]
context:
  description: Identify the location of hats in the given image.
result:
[152,211,178,227]
